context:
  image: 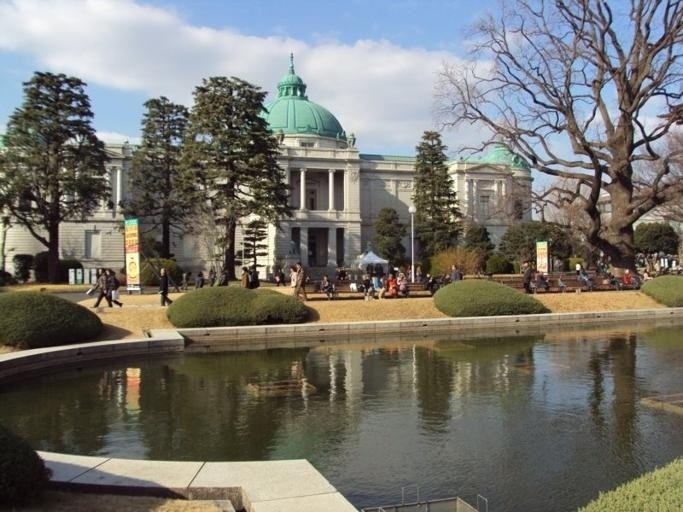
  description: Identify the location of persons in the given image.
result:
[523,257,657,294]
[294,262,307,301]
[171,268,228,292]
[320,275,335,301]
[159,268,172,305]
[240,266,250,287]
[105,270,123,308]
[92,268,112,308]
[276,269,286,286]
[450,263,463,282]
[289,266,299,288]
[247,266,258,289]
[360,264,450,303]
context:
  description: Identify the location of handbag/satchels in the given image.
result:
[86,288,96,296]
[111,289,120,301]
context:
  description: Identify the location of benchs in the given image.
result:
[299,281,366,300]
[394,283,433,298]
[495,276,642,294]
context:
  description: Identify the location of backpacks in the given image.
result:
[114,278,120,289]
[248,269,260,288]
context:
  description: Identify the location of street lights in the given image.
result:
[408,205,417,283]
